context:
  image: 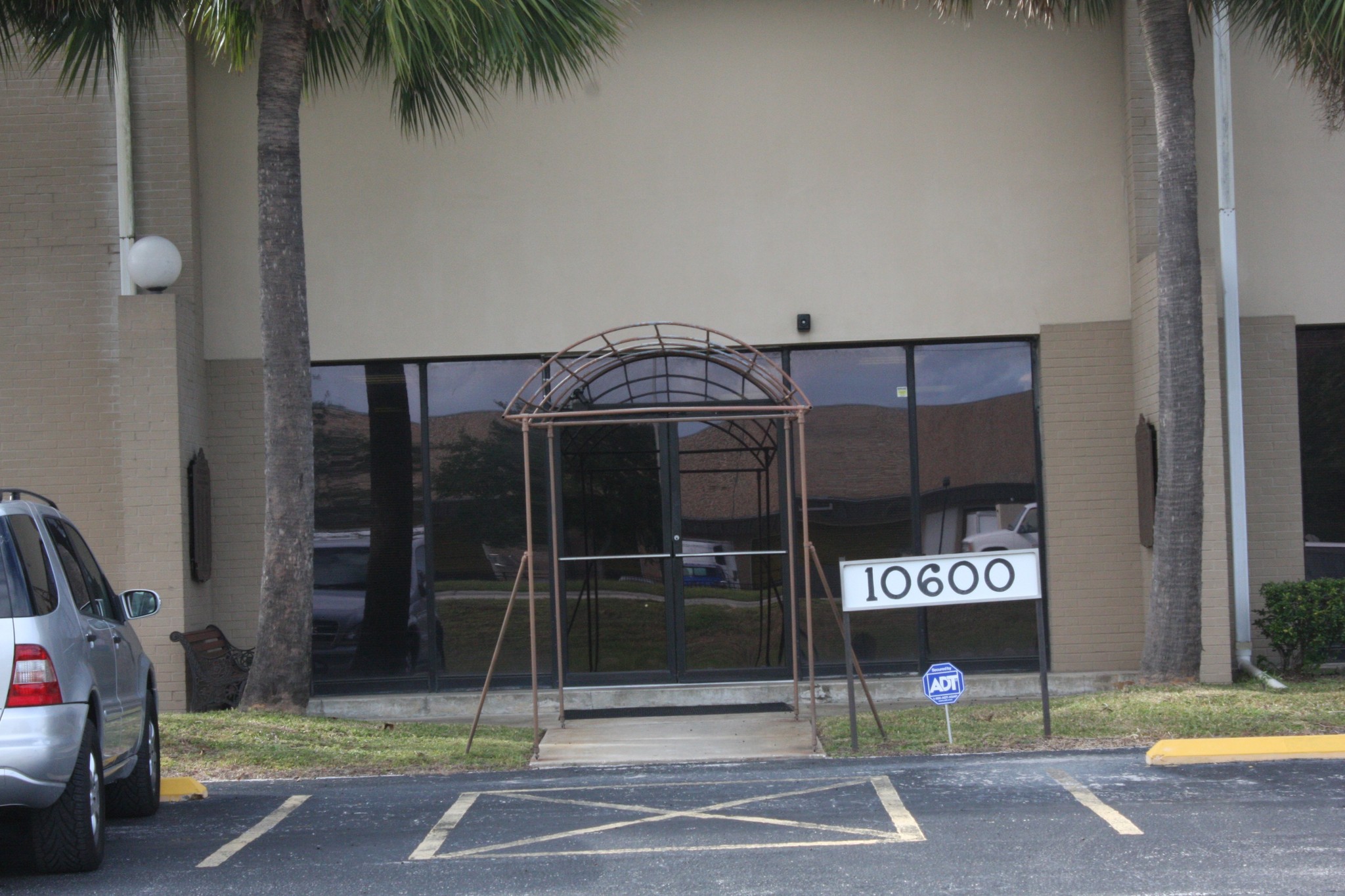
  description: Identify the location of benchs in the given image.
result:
[169,624,255,713]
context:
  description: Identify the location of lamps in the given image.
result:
[126,235,182,294]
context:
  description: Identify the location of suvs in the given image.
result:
[311,529,442,676]
[961,504,1039,553]
[0,488,167,869]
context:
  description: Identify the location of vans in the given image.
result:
[683,541,738,589]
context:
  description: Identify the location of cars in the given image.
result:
[683,563,731,589]
